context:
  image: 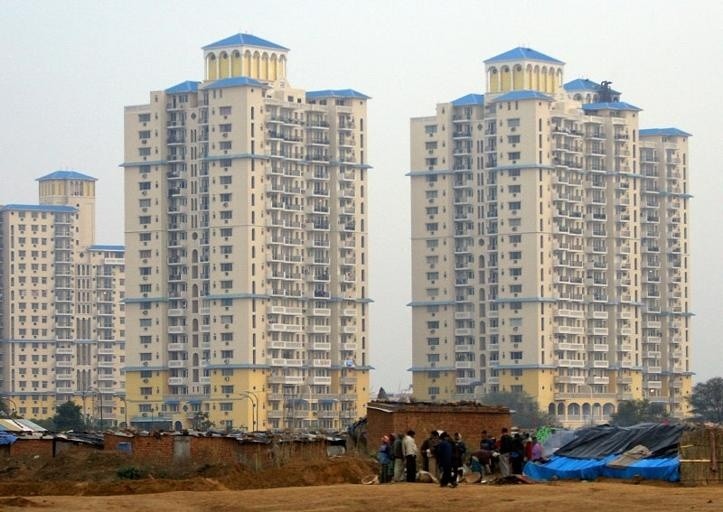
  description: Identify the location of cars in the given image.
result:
[510,426,519,437]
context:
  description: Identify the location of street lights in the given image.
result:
[245,390,258,432]
[0,397,16,411]
[112,394,128,427]
[87,386,104,432]
[332,398,340,432]
[240,394,255,432]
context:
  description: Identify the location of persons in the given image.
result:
[375,426,543,488]
